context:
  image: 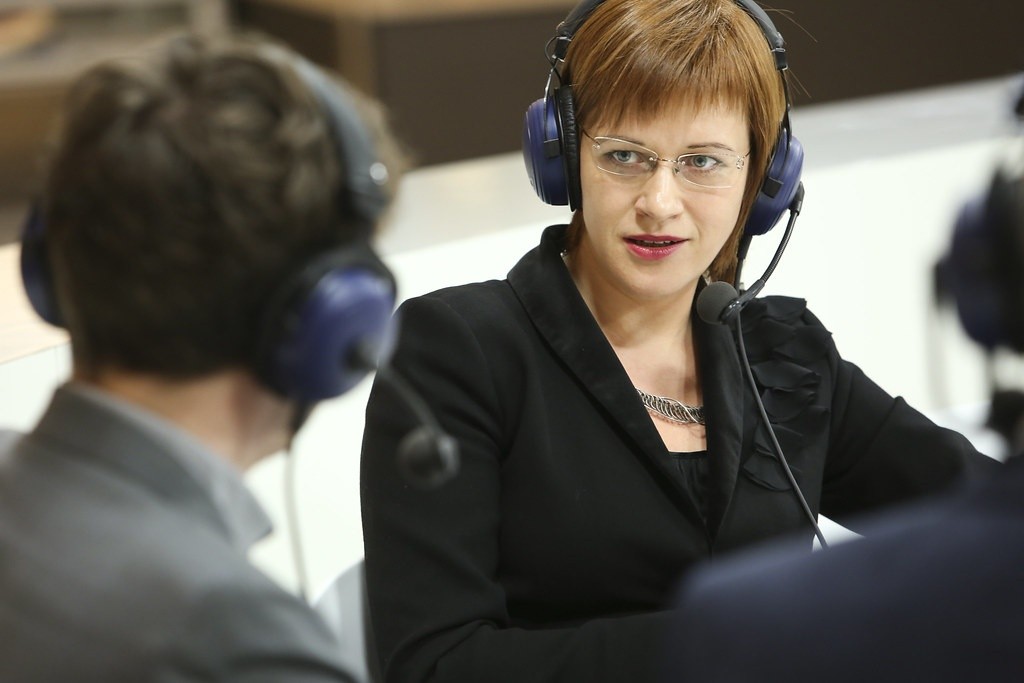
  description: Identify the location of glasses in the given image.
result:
[577,121,753,188]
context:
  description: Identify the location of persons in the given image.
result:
[360,0,1007,682]
[1,32,399,683]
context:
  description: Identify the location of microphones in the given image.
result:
[696,213,797,325]
[375,367,461,492]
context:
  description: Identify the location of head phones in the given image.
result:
[522,0,805,234]
[20,43,399,404]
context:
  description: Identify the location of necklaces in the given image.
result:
[637,388,706,426]
